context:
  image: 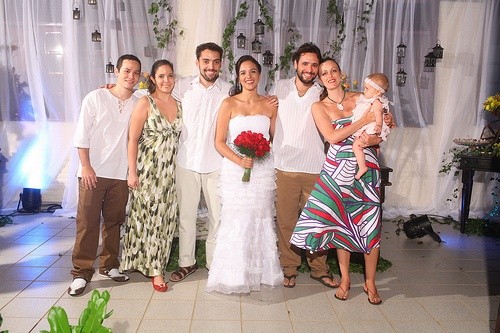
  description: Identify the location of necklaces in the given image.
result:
[328,90,346,111]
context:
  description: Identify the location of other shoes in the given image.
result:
[153,273,168,292]
[98,269,129,282]
[68,277,88,296]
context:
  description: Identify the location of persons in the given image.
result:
[351,73,390,181]
[311,58,392,305]
[68,55,141,295]
[123,60,183,291]
[266,42,341,289]
[206,53,284,293]
[96,41,279,281]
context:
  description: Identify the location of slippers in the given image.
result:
[282,267,298,288]
[309,272,340,288]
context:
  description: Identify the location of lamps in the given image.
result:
[397,43,406,64]
[16,188,42,213]
[92,24,102,42]
[88,0,96,5]
[106,56,114,74]
[251,19,273,67]
[403,213,441,242]
[396,69,407,87]
[423,44,444,72]
[237,33,246,49]
[73,8,80,19]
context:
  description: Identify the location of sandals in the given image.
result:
[170,261,209,282]
[362,281,383,306]
[334,281,351,301]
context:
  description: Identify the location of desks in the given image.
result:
[457,155,500,233]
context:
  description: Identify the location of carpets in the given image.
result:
[166,237,393,274]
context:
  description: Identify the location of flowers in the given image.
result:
[138,73,150,88]
[232,131,271,182]
[483,92,500,112]
